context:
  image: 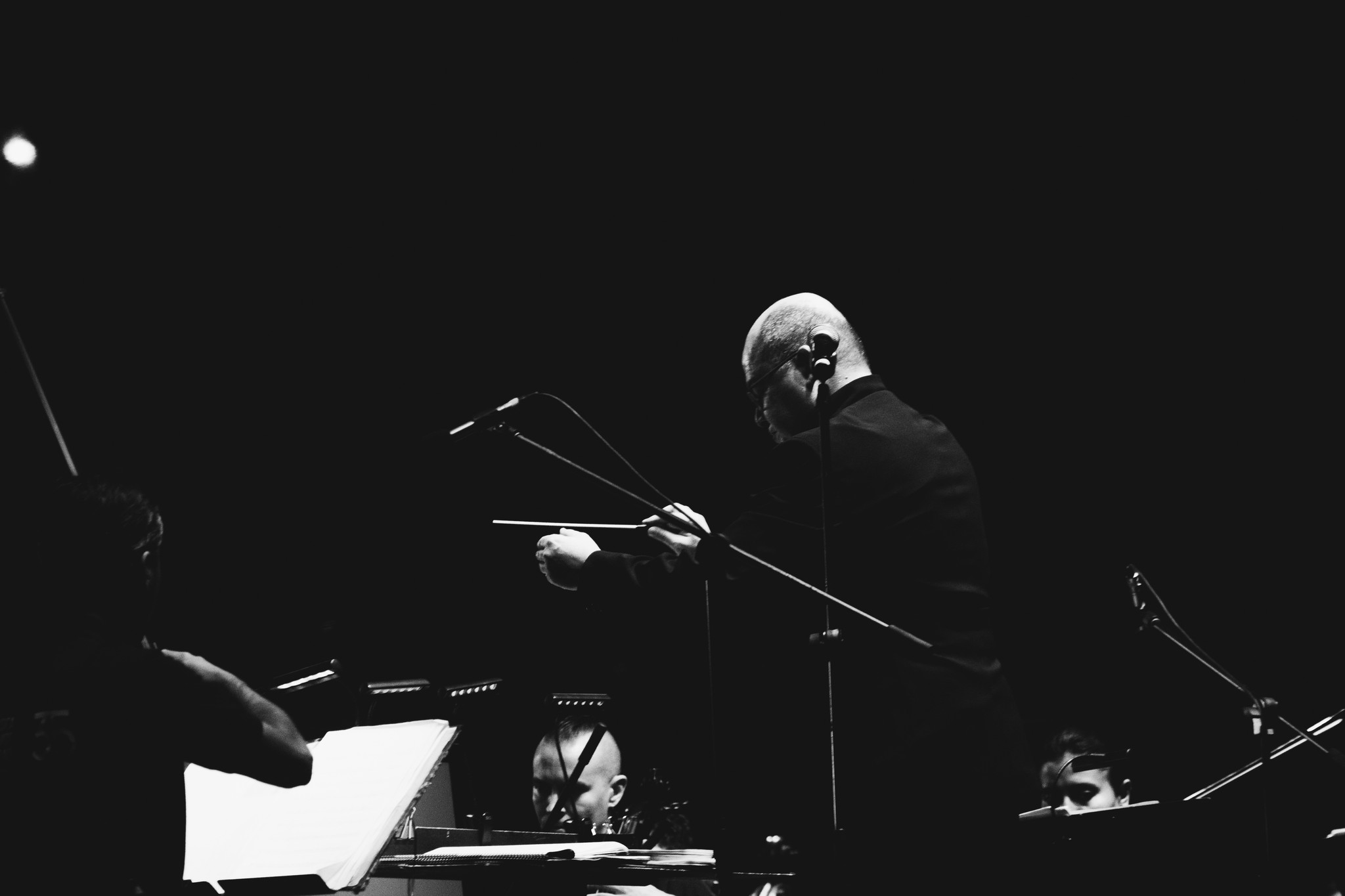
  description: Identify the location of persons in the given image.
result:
[0,291,1146,896]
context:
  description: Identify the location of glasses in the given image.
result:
[745,350,799,403]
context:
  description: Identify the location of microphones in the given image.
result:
[1126,570,1145,632]
[449,391,539,443]
[806,323,840,382]
